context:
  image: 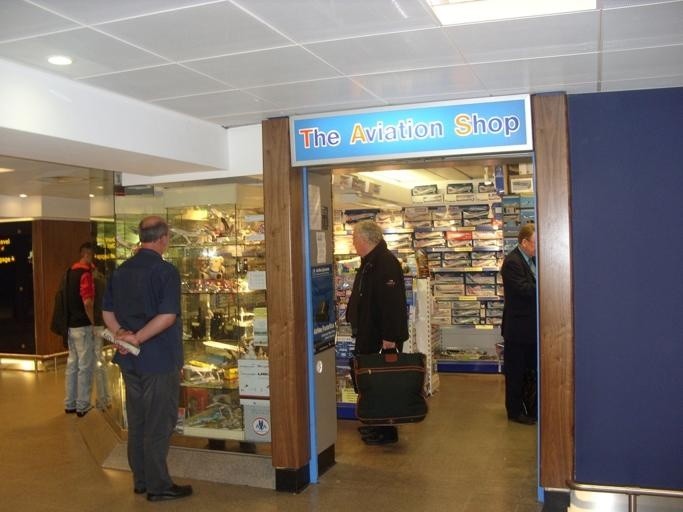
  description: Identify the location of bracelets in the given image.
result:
[116,327,125,335]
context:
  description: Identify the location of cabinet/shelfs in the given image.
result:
[333,198,505,420]
[163,203,266,440]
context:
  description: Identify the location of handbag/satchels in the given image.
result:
[349,344,429,427]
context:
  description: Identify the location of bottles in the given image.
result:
[192,306,224,340]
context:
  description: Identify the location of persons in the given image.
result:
[102,216,192,503]
[500,223,538,425]
[62,242,94,416]
[345,220,408,445]
[95,270,112,412]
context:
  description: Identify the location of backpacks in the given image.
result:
[50,267,70,338]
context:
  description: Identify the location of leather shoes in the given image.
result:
[357,425,399,446]
[507,407,538,425]
[64,401,94,418]
[134,480,193,502]
